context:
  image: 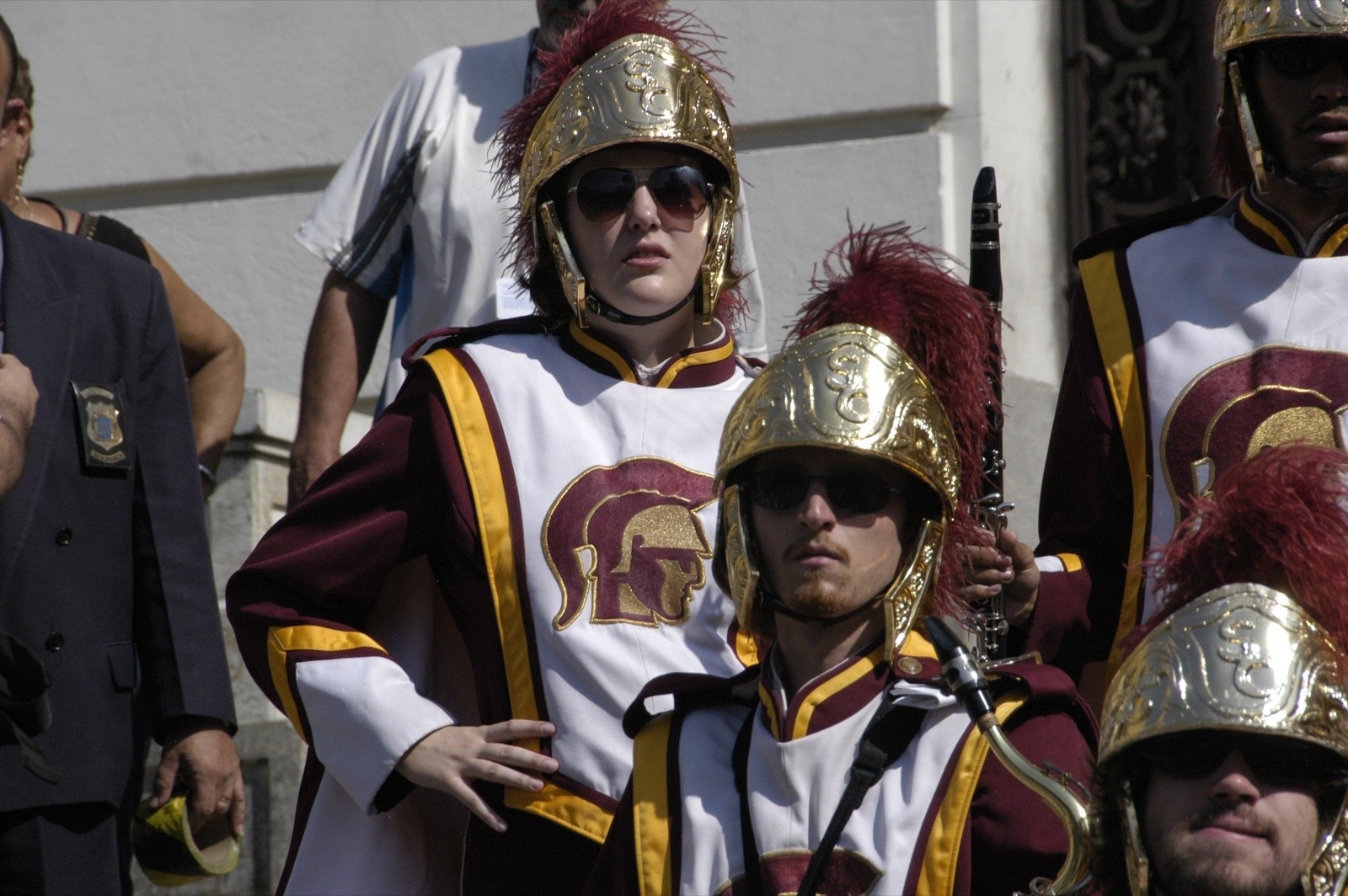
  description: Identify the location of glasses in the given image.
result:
[1155,733,1318,788]
[742,453,902,518]
[1261,38,1348,79]
[568,161,715,220]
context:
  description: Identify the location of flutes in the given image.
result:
[965,165,1015,661]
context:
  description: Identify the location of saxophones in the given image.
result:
[923,612,1111,896]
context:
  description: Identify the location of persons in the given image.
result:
[589,326,1097,896]
[224,0,776,896]
[0,16,244,896]
[938,0,1348,896]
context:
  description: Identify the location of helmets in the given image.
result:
[715,211,1014,661]
[493,0,739,322]
[1212,0,1348,198]
[1100,442,1347,896]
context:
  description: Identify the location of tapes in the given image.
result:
[130,794,241,888]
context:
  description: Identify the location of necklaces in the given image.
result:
[22,196,33,221]
[26,194,68,232]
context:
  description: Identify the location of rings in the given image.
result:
[219,796,232,806]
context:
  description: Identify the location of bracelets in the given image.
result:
[197,461,216,487]
